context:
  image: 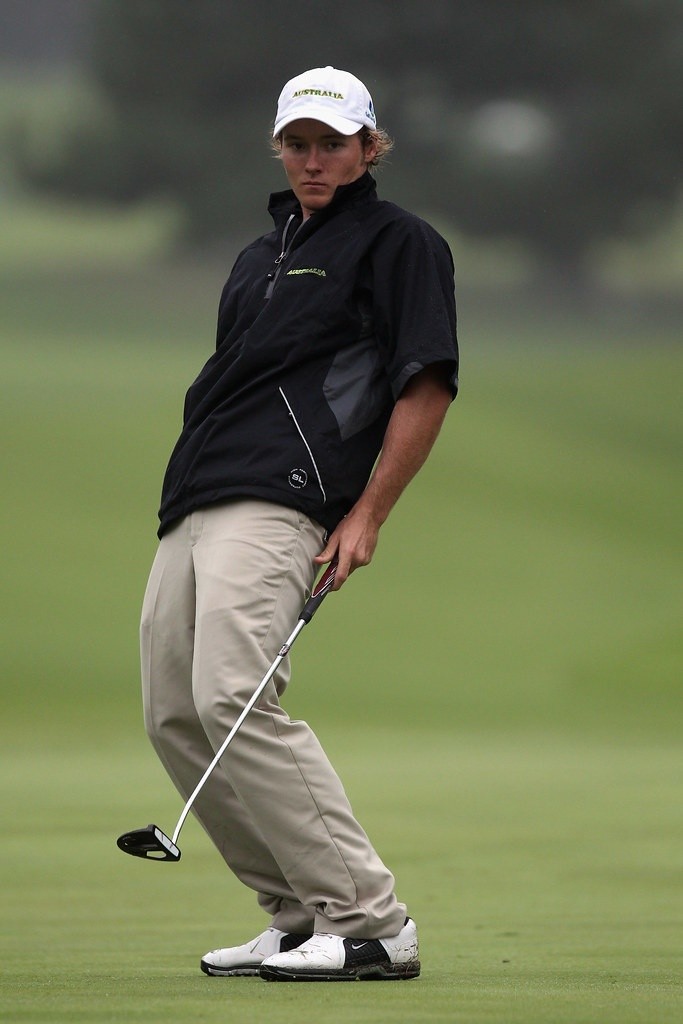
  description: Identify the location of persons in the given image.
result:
[141,66,460,982]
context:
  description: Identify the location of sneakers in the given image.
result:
[260,916,420,982]
[200,925,313,976]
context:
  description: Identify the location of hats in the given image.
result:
[273,66,377,135]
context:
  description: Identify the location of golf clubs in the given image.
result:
[116,554,341,862]
